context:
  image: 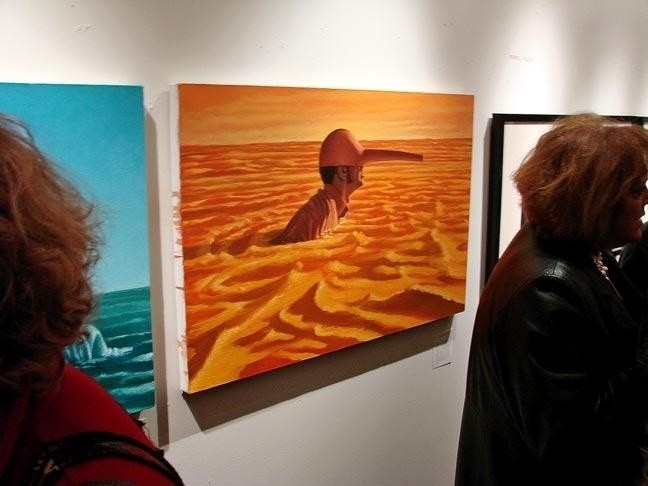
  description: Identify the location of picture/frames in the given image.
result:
[485,114,648,287]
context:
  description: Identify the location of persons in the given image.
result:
[452,109,647,486]
[267,129,425,244]
[1,105,190,485]
